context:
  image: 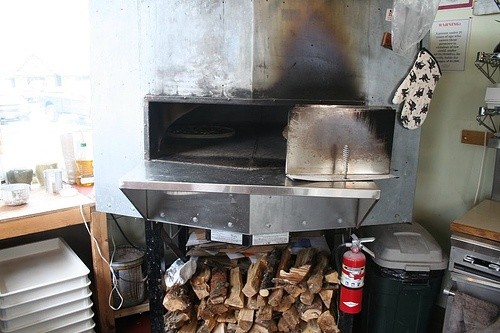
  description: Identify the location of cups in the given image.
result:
[44,168,62,195]
[35,163,58,187]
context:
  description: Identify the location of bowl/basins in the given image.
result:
[0,183,31,208]
[6,169,33,183]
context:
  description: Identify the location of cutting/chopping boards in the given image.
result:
[450,198,500,240]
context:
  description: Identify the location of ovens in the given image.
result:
[88,0,430,235]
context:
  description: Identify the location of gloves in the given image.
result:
[392,40,442,130]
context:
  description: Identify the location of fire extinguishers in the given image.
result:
[336,234,375,315]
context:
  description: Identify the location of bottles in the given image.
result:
[74,142,95,187]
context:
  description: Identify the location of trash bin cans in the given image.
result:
[363,223,450,333]
